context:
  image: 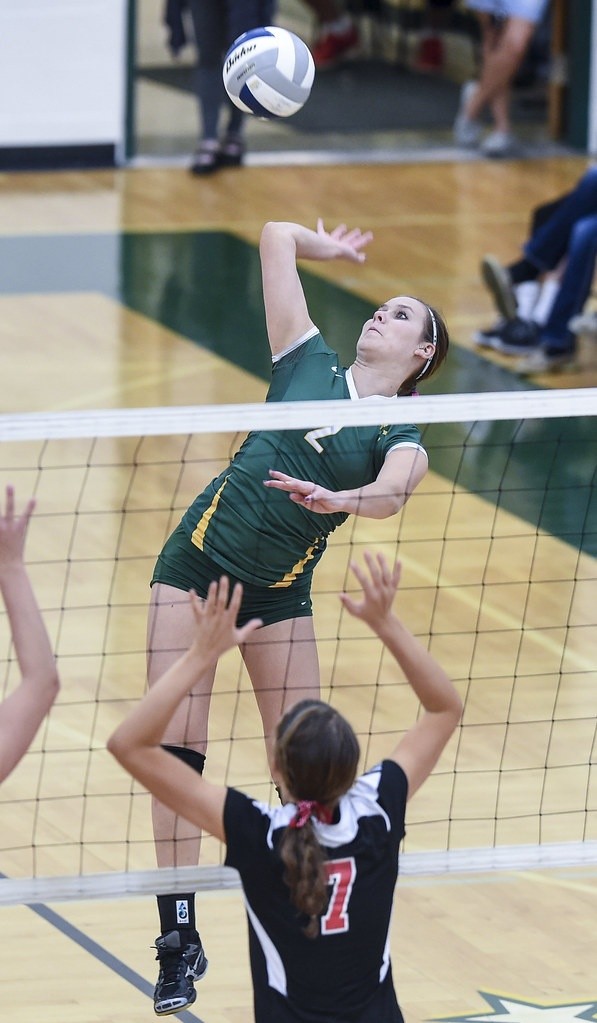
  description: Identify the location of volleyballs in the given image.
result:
[222,26,316,118]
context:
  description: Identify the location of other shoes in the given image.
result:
[192,148,218,173]
[216,134,246,166]
[482,130,516,156]
[513,338,580,377]
[453,79,483,145]
[474,319,543,354]
[482,256,518,316]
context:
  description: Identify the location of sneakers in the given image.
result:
[150,930,208,1015]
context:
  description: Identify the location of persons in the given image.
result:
[473,154,597,375]
[448,1,556,153]
[105,550,465,1023]
[145,216,450,1017]
[149,0,280,178]
[1,486,63,788]
